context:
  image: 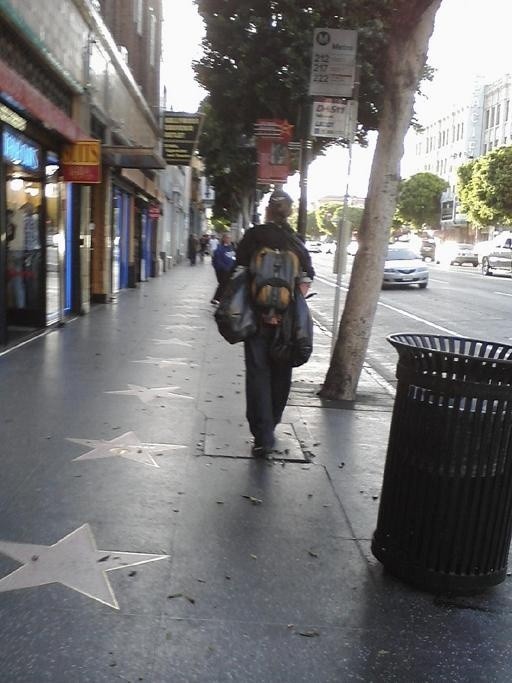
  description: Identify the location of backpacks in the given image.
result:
[214,233,312,367]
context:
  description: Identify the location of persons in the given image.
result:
[208,232,238,307]
[229,188,316,450]
[208,234,220,256]
[185,232,199,267]
[198,234,208,263]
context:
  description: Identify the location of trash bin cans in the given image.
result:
[371,332,512,592]
